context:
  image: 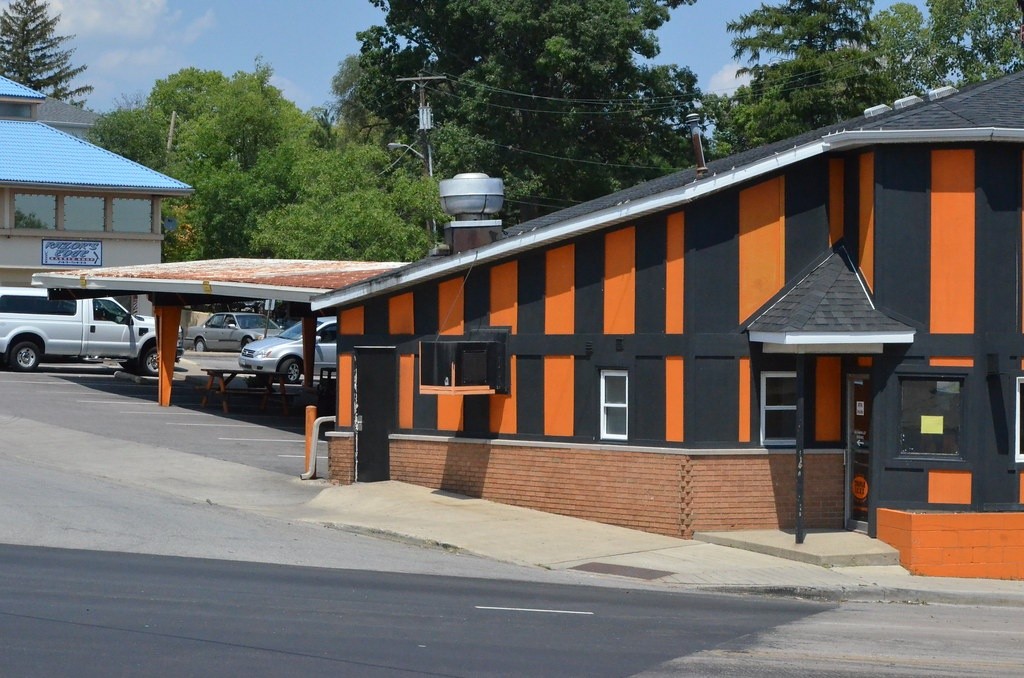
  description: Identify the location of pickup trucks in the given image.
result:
[0,285,188,377]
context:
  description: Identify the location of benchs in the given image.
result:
[196,386,300,397]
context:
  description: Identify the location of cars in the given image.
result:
[239,316,341,384]
[185,310,287,351]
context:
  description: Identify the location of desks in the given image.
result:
[201,369,293,413]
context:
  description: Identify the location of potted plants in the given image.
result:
[241,375,276,404]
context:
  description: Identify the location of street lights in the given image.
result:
[388,141,435,254]
